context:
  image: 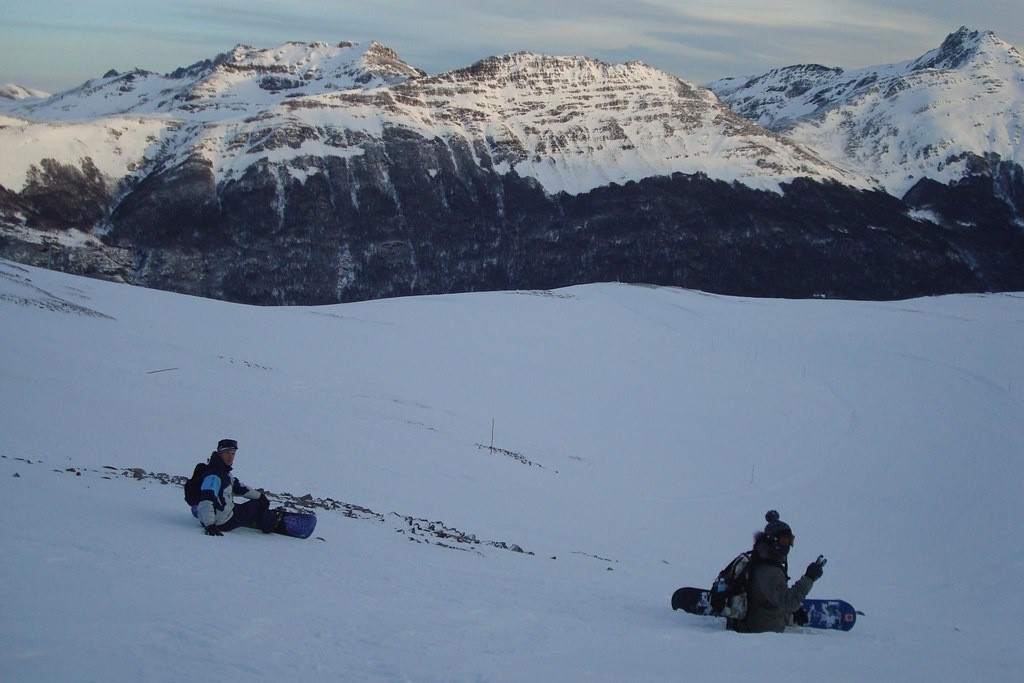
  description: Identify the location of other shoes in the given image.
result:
[271,506,283,530]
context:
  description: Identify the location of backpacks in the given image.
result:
[708,551,786,620]
[184,463,224,506]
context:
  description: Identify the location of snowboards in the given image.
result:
[191,505,318,540]
[672,587,856,632]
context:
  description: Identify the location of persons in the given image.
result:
[197,439,283,537]
[726,510,827,634]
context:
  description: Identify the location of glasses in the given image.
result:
[791,536,795,547]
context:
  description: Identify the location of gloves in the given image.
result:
[204,524,223,536]
[805,555,827,581]
[259,494,269,508]
[793,606,808,627]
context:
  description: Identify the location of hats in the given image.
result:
[217,439,237,453]
[764,510,793,538]
